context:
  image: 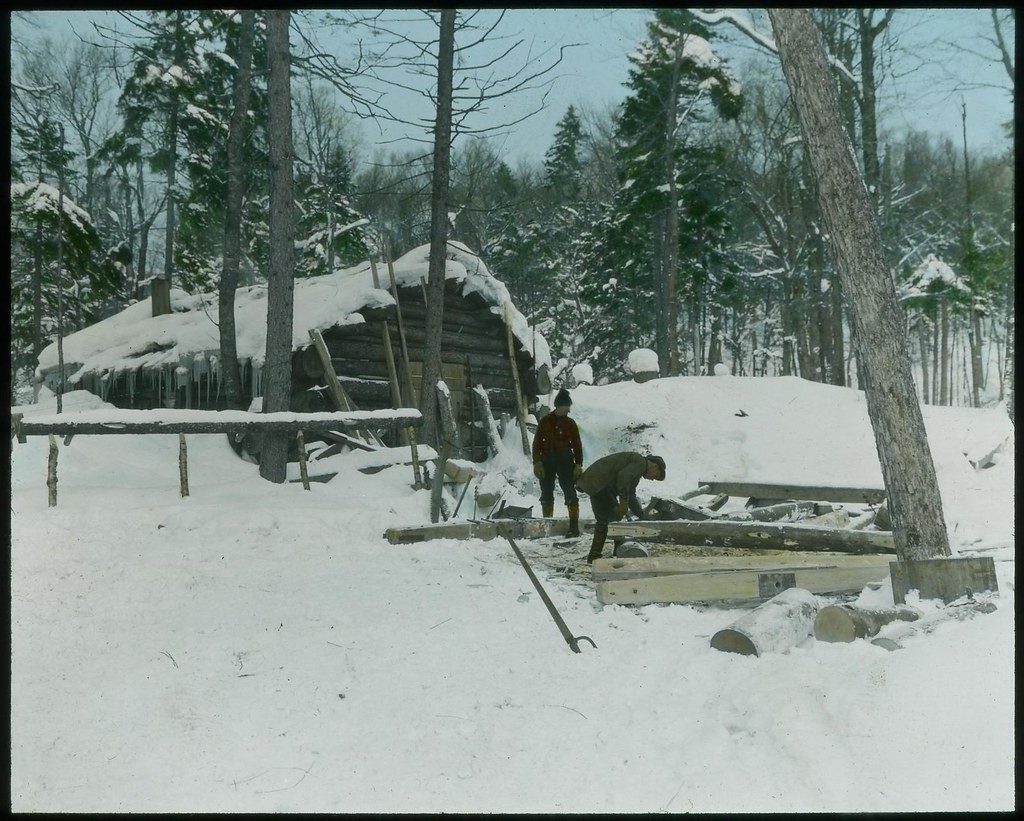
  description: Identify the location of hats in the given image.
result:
[554,390,573,407]
[647,454,666,481]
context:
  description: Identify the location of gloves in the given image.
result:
[533,461,545,480]
[616,497,629,516]
[573,463,582,481]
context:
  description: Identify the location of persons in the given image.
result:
[574,452,666,565]
[532,389,583,539]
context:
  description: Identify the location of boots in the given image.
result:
[565,503,580,538]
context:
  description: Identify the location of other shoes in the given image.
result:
[588,550,603,564]
